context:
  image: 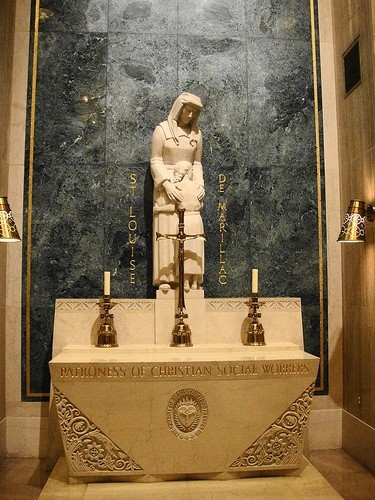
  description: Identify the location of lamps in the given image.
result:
[0,196,21,243]
[337,199,375,243]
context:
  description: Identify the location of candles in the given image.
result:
[104,271,110,295]
[252,269,258,293]
